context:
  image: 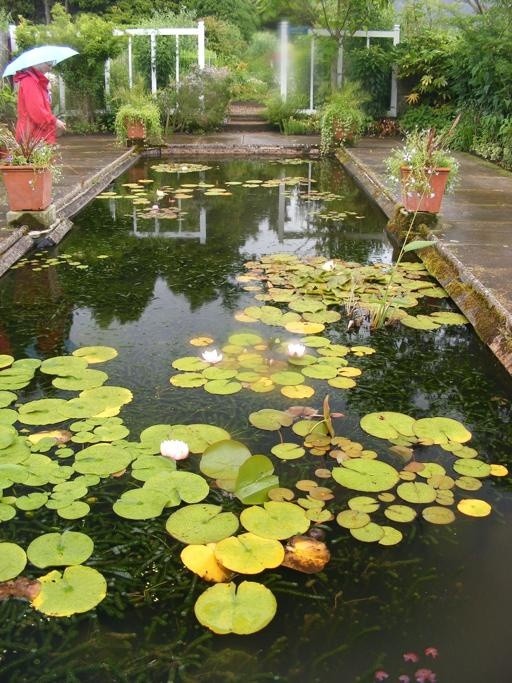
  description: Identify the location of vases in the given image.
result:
[0,163,52,211]
[401,168,448,214]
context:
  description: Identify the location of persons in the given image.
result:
[15,61,66,159]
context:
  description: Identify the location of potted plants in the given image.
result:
[320,80,371,156]
[105,73,163,145]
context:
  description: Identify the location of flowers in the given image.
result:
[384,127,461,198]
[0,113,66,190]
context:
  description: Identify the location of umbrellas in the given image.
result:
[1,44,80,78]
